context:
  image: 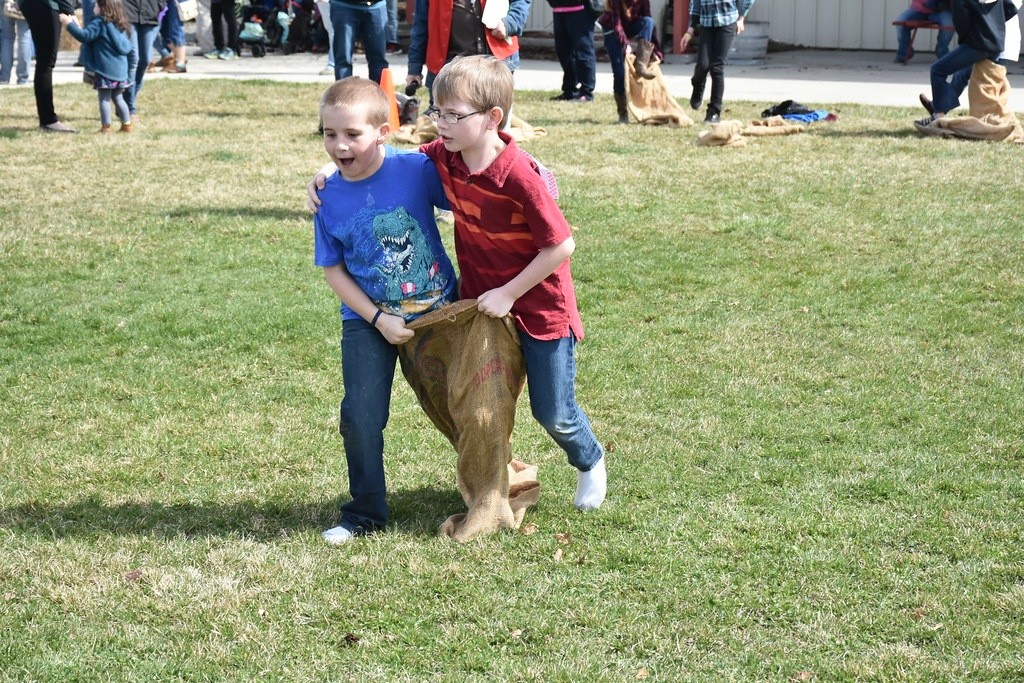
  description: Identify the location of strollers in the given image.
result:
[236,3,280,57]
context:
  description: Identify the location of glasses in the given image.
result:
[423,107,492,124]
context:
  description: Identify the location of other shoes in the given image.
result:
[919,94,931,112]
[320,67,335,75]
[219,48,239,60]
[914,116,935,130]
[167,66,186,72]
[98,126,112,133]
[705,108,720,122]
[205,48,219,59]
[551,93,570,101]
[119,124,132,133]
[690,90,703,109]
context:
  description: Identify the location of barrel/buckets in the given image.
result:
[727,21,769,59]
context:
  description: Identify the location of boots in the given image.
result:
[635,39,655,80]
[615,92,628,125]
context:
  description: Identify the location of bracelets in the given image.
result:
[371,310,383,326]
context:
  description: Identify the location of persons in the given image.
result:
[601,0,663,125]
[891,0,955,64]
[116,0,188,122]
[406,0,533,106]
[547,0,604,101]
[307,55,608,512]
[0,0,32,85]
[680,0,756,123]
[59,0,139,134]
[193,0,329,60]
[17,0,80,134]
[328,0,399,85]
[914,0,1019,131]
[315,76,458,545]
[73,0,96,67]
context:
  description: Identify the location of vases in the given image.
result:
[726,21,770,59]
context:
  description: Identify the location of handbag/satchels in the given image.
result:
[176,0,199,22]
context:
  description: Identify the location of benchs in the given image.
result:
[890,20,938,66]
